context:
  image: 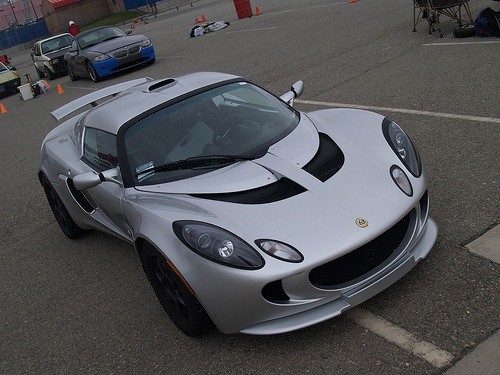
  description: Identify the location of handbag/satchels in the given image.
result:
[474,7,500,38]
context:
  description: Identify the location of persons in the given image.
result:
[68,21,80,36]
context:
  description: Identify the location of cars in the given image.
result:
[31,32,74,81]
[0,61,21,99]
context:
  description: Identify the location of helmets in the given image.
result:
[69,21,74,25]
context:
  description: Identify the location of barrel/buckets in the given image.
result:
[17,83,33,101]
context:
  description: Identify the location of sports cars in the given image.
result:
[63,25,156,83]
[37,70,440,340]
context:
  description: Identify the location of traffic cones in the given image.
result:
[351,0,359,3]
[255,7,262,16]
[0,103,8,113]
[58,84,64,95]
[45,80,50,89]
[195,18,204,23]
[202,14,206,22]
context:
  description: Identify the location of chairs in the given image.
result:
[42,46,49,53]
[59,41,67,48]
[411,0,474,38]
